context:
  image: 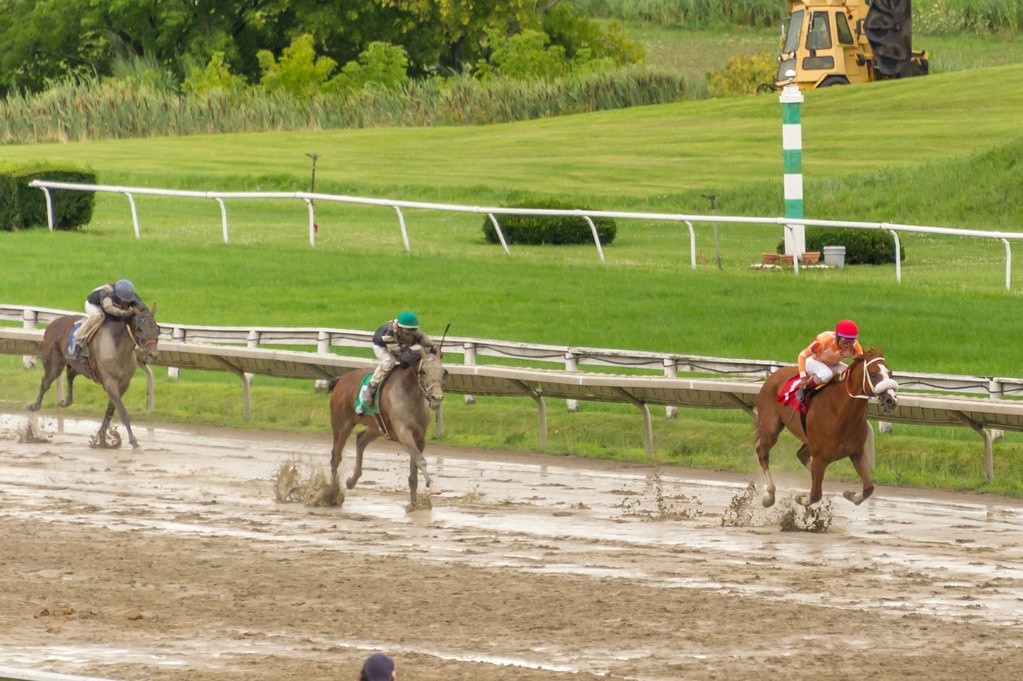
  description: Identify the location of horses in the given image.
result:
[26,301,160,453]
[751,345,900,508]
[327,343,449,509]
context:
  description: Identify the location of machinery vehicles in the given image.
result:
[753,0,929,96]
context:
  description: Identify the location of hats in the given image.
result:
[363,652,394,681]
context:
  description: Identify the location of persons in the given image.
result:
[360,653,396,680]
[365,310,441,405]
[796,319,864,404]
[69,279,140,359]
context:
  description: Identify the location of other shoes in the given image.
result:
[795,389,807,406]
[361,388,376,407]
[73,345,83,362]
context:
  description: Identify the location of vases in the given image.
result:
[760,245,847,270]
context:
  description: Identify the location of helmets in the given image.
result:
[113,279,135,303]
[398,311,419,328]
[836,320,859,340]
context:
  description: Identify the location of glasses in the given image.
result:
[398,327,417,335]
[839,336,855,344]
